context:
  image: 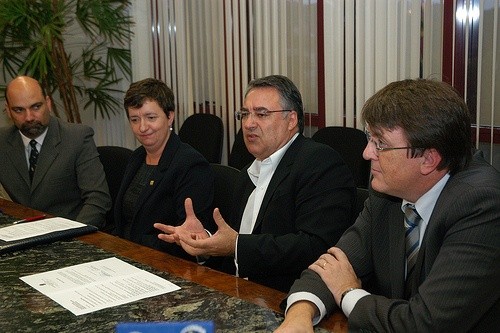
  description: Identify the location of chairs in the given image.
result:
[310,126,371,190]
[96,147,133,232]
[178,113,255,170]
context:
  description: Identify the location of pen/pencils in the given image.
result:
[12,214,46,224]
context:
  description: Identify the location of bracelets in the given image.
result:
[340,286,362,309]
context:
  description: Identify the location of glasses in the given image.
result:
[363,126,423,157]
[236,109,292,121]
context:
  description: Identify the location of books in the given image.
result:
[0,224,98,254]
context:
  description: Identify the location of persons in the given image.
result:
[154,75,358,295]
[0,76,113,229]
[273,79,500,333]
[99,78,215,263]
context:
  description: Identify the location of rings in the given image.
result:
[321,260,328,268]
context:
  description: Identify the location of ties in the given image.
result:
[29,140,39,185]
[402,204,421,301]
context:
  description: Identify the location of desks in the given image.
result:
[0,199,348,332]
